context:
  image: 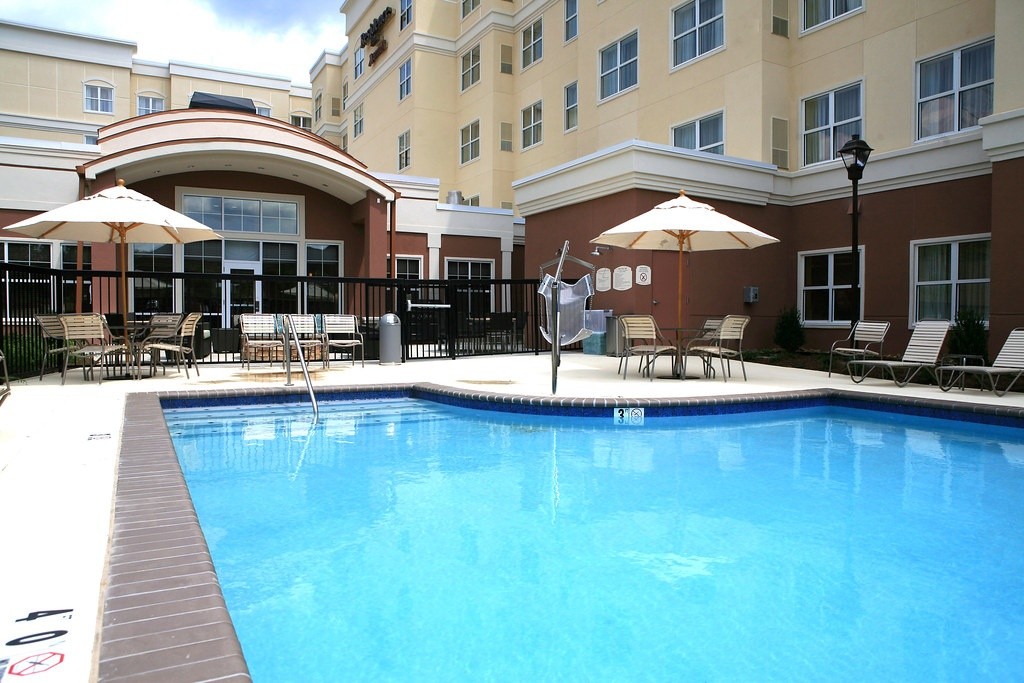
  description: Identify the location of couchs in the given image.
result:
[164,313,239,359]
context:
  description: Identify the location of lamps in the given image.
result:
[590,246,609,255]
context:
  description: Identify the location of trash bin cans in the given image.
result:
[604,315,633,357]
[378,312,402,364]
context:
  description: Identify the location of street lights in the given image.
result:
[837,132,875,348]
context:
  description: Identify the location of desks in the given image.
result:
[655,328,716,379]
[466,318,517,353]
[940,352,985,391]
[103,326,168,380]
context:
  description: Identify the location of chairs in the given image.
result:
[829,320,890,379]
[31,313,202,384]
[437,309,529,354]
[847,321,950,388]
[242,419,276,440]
[240,314,363,370]
[935,327,1024,397]
[618,314,747,383]
[289,420,311,437]
[324,418,357,435]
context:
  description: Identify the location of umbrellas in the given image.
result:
[3,179,224,380]
[589,190,781,379]
